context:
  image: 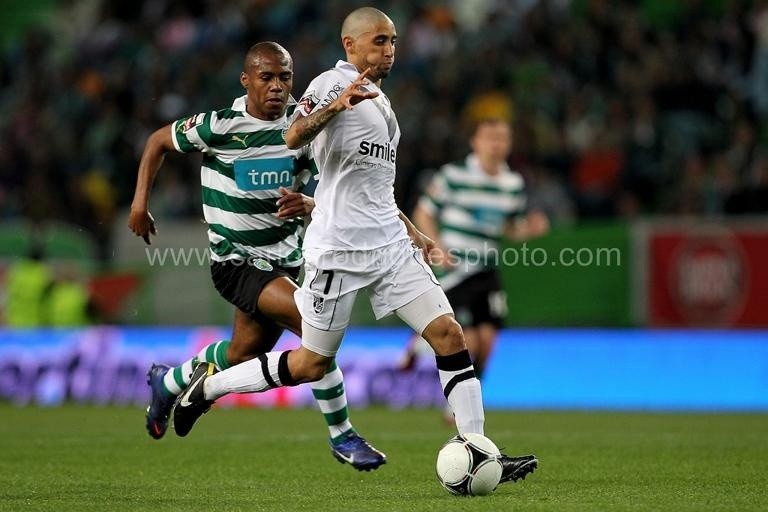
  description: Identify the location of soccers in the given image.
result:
[435,434,504,495]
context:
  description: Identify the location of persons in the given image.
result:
[0,0,768,330]
[125,41,389,474]
[392,117,548,426]
[170,6,538,488]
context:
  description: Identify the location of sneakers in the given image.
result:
[145,363,172,439]
[500,455,537,483]
[328,435,386,471]
[174,361,221,437]
